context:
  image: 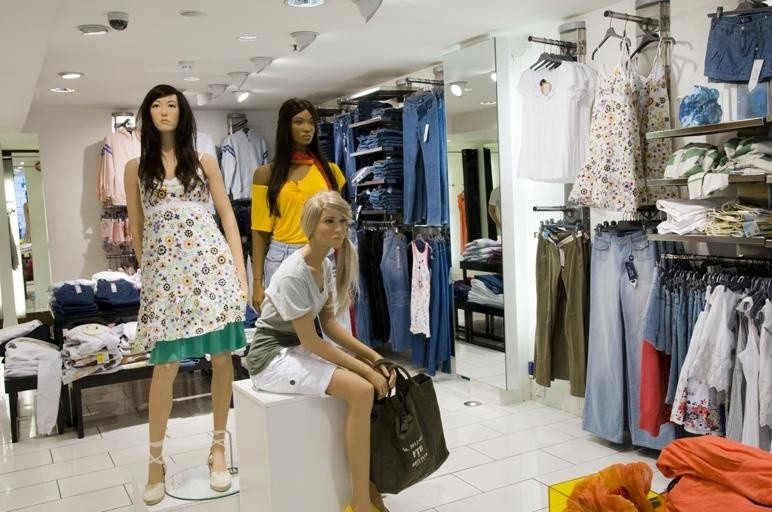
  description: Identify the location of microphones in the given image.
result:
[231,377,348,512]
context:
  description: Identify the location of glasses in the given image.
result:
[448,82,469,97]
[197,0,383,106]
[78,24,110,36]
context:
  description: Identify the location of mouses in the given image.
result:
[703,255,772,327]
[520,34,581,79]
[224,114,266,144]
[102,120,143,145]
[589,10,677,61]
[406,225,434,253]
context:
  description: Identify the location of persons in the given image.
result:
[122,84,253,506]
[22,201,31,244]
[244,189,401,512]
[248,97,356,365]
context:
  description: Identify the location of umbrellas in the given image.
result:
[208,452,232,492]
[144,463,167,505]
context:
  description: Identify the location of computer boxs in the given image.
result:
[106,11,128,31]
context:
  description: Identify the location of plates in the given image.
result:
[48,299,243,438]
[453,258,505,352]
[641,118,771,245]
[337,80,425,215]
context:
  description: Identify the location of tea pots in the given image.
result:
[442,33,512,396]
[0,133,54,326]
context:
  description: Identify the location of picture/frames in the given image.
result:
[5,311,73,443]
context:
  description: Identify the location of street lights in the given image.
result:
[370,358,449,494]
